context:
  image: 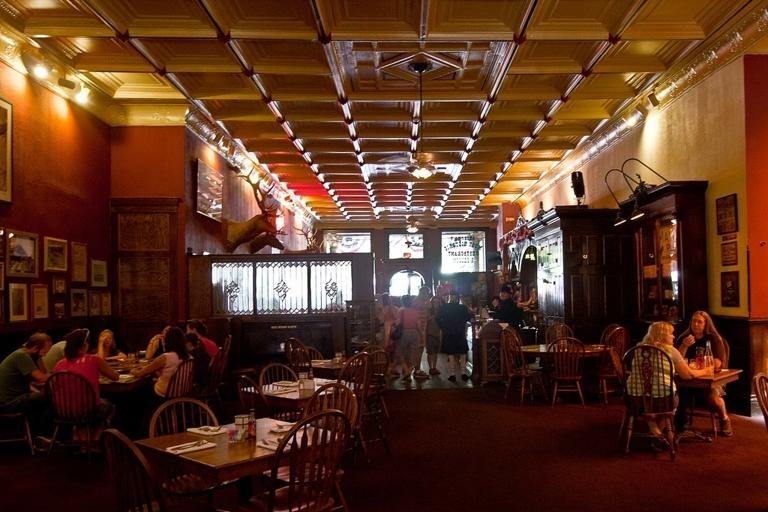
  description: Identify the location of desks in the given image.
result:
[671,368,742,437]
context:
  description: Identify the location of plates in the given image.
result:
[119,374,133,378]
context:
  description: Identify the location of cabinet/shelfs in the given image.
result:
[527,206,636,328]
[618,181,709,343]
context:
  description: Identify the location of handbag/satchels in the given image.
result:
[391,327,401,339]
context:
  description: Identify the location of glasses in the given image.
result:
[73,329,90,342]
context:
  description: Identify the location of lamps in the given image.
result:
[59,69,75,89]
[405,62,436,179]
[406,181,419,234]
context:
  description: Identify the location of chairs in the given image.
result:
[475,315,627,405]
[753,372,768,428]
[684,335,728,438]
[622,345,678,459]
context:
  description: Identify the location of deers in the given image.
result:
[250,226,289,255]
[228,164,277,253]
[284,223,325,255]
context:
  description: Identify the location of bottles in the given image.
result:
[309,367,315,379]
[694,341,715,369]
[335,352,342,363]
[248,409,257,440]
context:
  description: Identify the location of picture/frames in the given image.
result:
[0,97,12,203]
[0,227,112,322]
[720,271,739,307]
[720,241,738,266]
[196,155,224,224]
[715,193,738,235]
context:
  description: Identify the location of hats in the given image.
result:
[500,287,512,294]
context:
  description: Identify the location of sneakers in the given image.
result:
[392,368,468,383]
[721,417,731,433]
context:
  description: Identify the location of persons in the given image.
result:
[487,283,534,330]
[436,291,475,383]
[379,287,441,384]
[676,310,733,437]
[0,318,221,455]
[624,322,694,448]
